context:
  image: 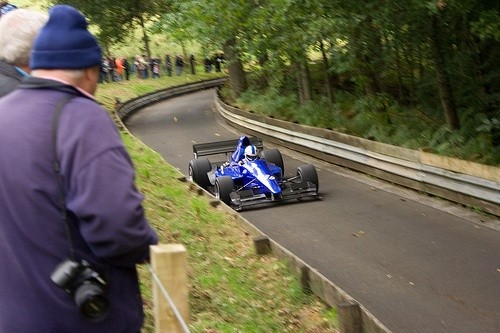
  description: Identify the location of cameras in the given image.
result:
[50,258,112,322]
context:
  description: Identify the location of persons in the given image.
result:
[165,54,172,76]
[175,55,183,75]
[189,54,195,74]
[0,0,157,333]
[238,145,260,165]
[204,53,225,72]
[150,56,160,78]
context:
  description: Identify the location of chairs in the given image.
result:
[230,135,260,167]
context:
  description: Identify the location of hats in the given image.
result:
[30,4,102,69]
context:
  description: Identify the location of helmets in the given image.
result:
[244,144,259,162]
